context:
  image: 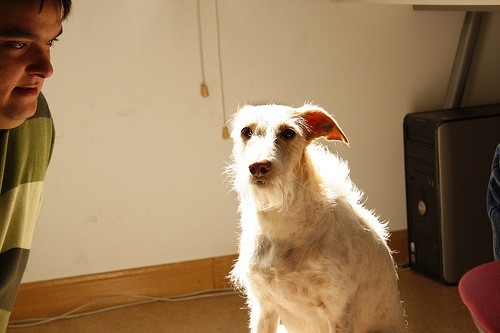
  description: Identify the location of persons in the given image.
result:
[0,0,74,333]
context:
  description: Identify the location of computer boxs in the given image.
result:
[403,103,500,285]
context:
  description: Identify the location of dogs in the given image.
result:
[224,102,409,333]
